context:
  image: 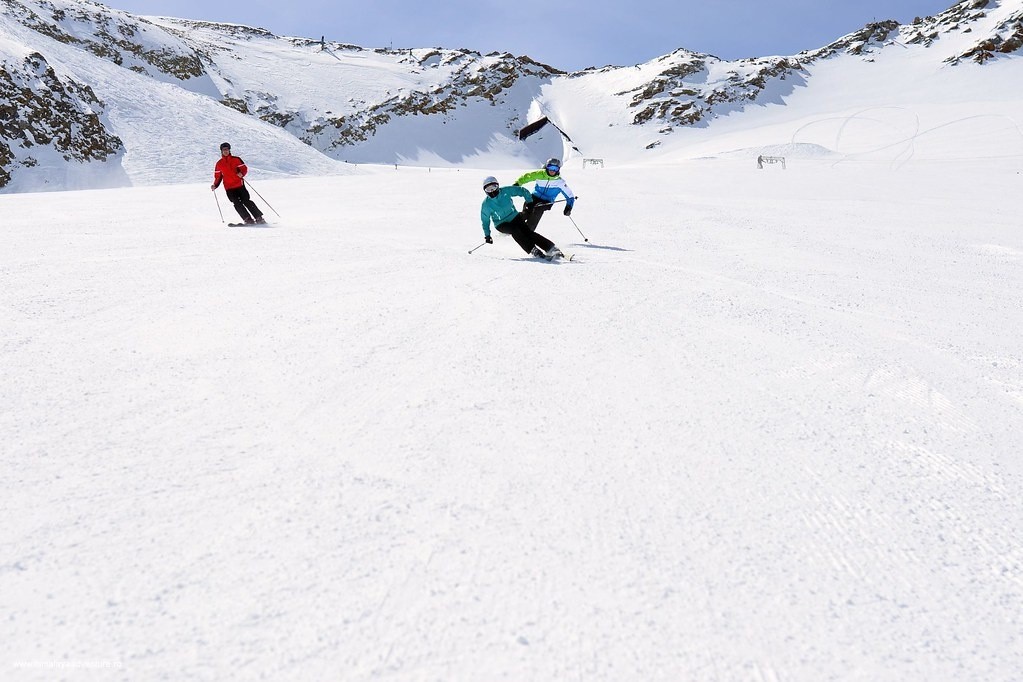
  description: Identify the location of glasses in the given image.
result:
[548,165,559,172]
[484,184,499,193]
[222,148,229,151]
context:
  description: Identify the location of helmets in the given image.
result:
[546,158,561,168]
[220,143,230,149]
[482,176,498,194]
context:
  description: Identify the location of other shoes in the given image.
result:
[245,217,253,224]
[531,247,545,258]
[256,216,266,224]
[551,248,564,259]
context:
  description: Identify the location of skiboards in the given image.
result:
[228,221,276,226]
[544,253,575,262]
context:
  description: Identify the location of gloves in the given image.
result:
[527,203,533,213]
[485,236,492,243]
[564,205,572,216]
[512,183,518,187]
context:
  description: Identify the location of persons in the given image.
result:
[211,142,266,225]
[758,155,766,169]
[512,158,575,232]
[481,176,565,261]
